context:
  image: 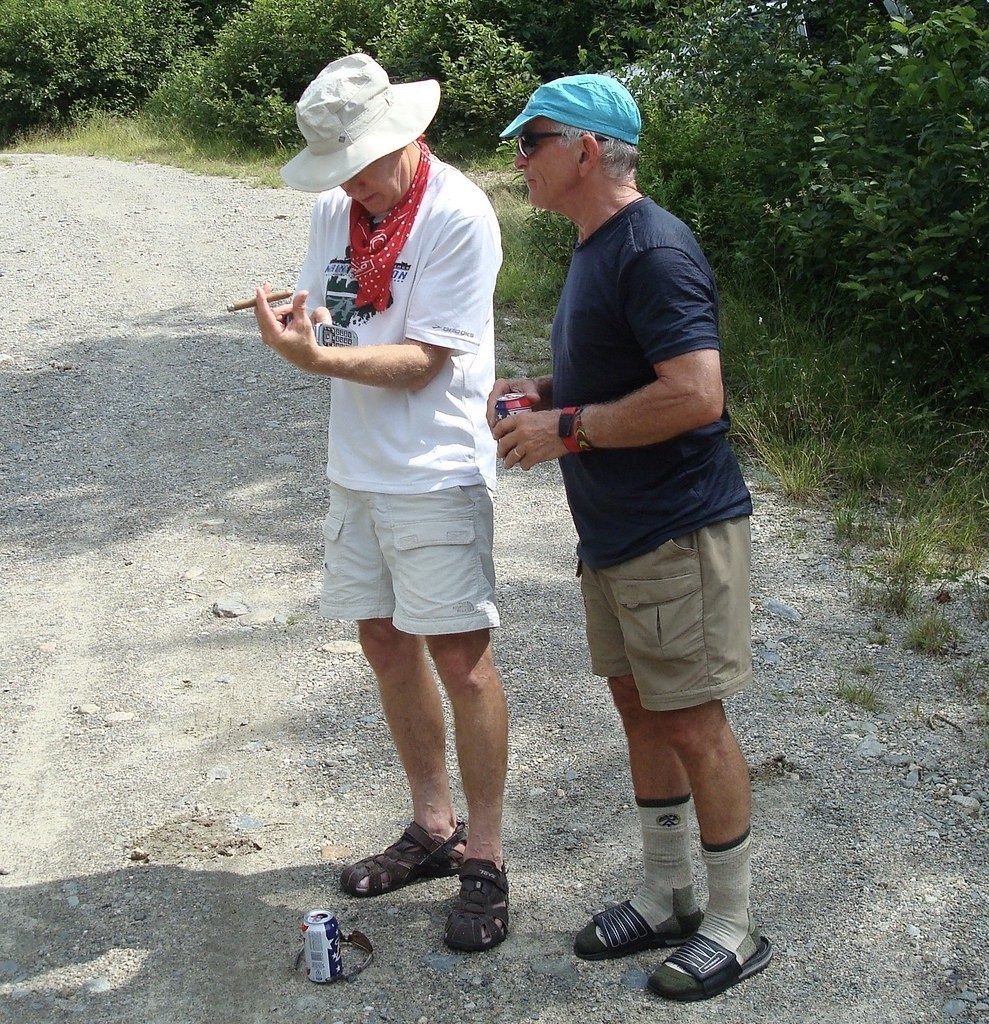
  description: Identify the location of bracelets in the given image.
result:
[559,407,590,452]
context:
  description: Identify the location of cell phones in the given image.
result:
[285,314,359,347]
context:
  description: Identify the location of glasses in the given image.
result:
[517,133,611,159]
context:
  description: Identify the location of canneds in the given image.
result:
[494,392,533,466]
[301,909,342,984]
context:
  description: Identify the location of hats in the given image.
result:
[279,53,441,193]
[500,73,643,148]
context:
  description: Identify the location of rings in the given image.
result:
[514,449,521,457]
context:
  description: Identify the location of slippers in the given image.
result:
[650,927,774,1001]
[575,903,706,962]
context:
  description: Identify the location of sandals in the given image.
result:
[443,855,511,950]
[343,821,469,898]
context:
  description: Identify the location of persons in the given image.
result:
[255,54,512,952]
[486,74,773,1001]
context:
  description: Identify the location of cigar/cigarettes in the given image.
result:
[228,290,294,312]
